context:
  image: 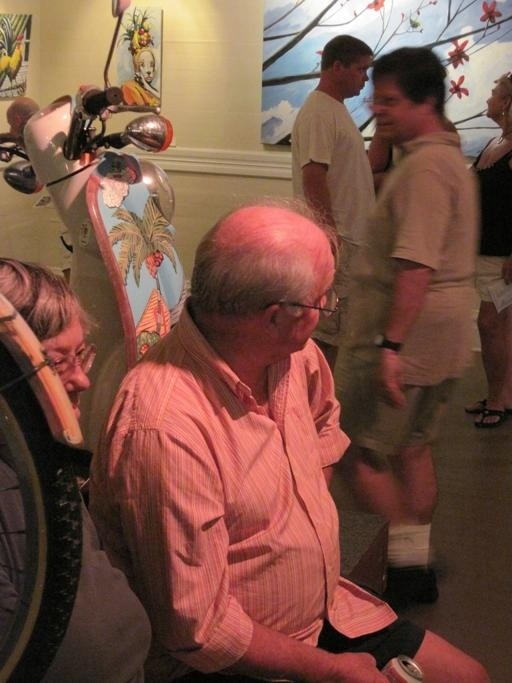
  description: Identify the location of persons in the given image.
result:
[291,34,375,351]
[466,71,512,429]
[0,254,153,682]
[331,47,478,610]
[94,195,490,683]
[363,103,462,196]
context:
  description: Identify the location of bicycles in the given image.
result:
[0,253,94,680]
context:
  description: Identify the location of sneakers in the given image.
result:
[382,564,440,615]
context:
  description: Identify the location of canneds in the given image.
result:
[381,654,423,683]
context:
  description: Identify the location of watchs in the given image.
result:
[373,333,402,352]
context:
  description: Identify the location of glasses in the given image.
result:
[53,343,99,376]
[258,281,341,318]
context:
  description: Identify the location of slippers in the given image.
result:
[464,397,512,428]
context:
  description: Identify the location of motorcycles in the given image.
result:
[0,1,188,457]
